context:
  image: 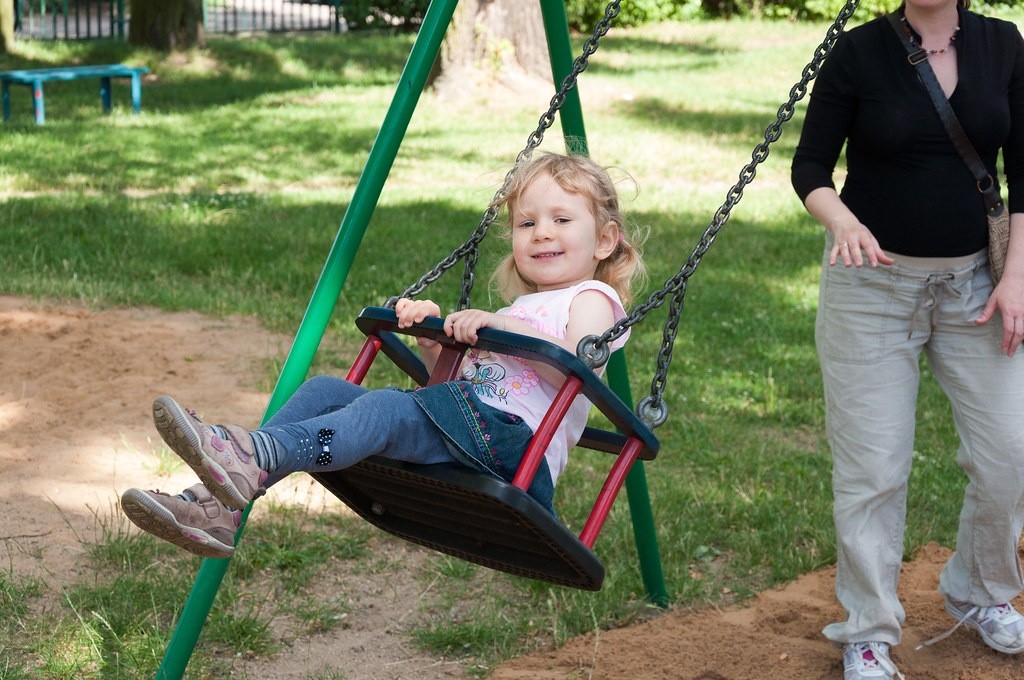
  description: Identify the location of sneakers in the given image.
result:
[840,640,896,680]
[942,592,1023,654]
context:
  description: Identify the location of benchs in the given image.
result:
[0,63,149,128]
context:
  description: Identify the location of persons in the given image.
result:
[121,151,650,558]
[791,1,1022,679]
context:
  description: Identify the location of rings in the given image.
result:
[839,243,847,248]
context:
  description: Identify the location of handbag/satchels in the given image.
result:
[985,185,1008,285]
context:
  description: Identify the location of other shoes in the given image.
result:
[154,394,266,509]
[120,483,243,557]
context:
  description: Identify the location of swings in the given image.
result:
[306,0,864,593]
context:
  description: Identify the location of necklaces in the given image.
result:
[900,6,959,55]
